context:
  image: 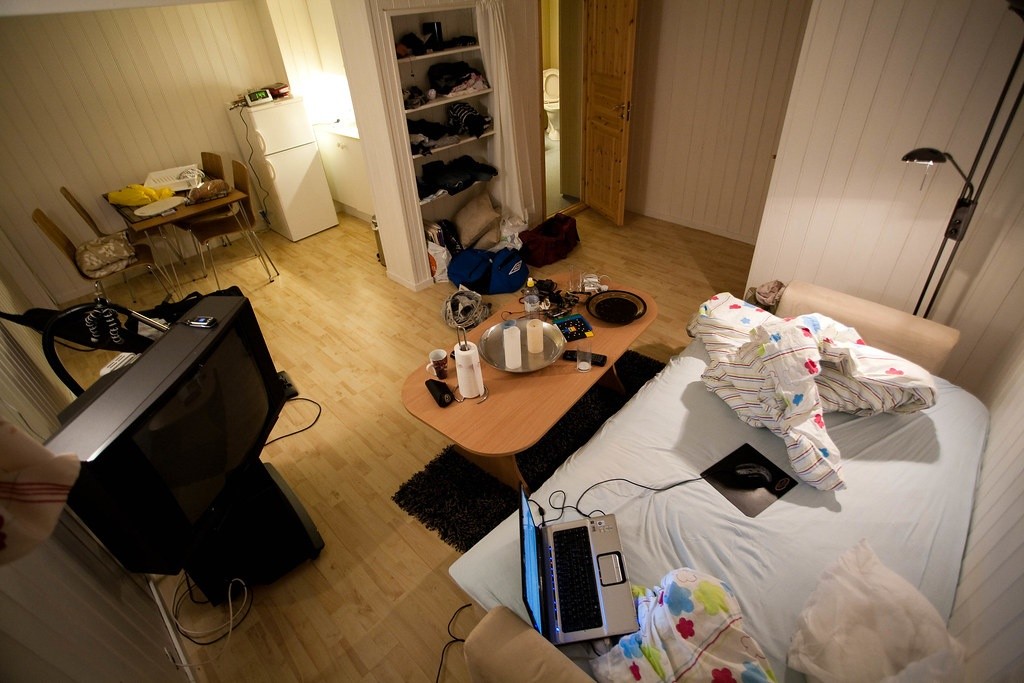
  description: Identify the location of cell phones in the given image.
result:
[189,316,218,328]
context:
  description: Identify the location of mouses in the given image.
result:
[734,463,773,483]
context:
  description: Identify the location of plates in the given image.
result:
[585,290,648,323]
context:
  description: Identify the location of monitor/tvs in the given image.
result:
[40,295,290,576]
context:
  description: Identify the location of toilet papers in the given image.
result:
[527,319,544,353]
[454,342,484,399]
[504,326,522,369]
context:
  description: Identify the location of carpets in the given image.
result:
[390,352,667,555]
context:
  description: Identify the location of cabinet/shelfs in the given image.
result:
[332,0,530,293]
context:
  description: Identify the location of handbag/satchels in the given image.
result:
[444,290,489,326]
[447,248,531,297]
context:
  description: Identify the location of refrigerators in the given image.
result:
[226,91,339,242]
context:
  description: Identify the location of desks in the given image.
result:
[402,269,657,491]
[100,173,277,304]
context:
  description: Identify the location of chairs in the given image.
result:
[32,152,279,316]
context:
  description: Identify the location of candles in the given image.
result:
[504,326,521,368]
[527,319,543,353]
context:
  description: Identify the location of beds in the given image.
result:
[448,281,991,683]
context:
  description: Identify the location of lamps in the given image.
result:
[902,147,978,318]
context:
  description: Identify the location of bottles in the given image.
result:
[524,277,540,319]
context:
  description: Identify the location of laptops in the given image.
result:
[518,481,642,648]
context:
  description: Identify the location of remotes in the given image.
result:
[562,349,607,366]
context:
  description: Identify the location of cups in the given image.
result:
[568,264,582,292]
[576,339,591,372]
[425,349,448,380]
